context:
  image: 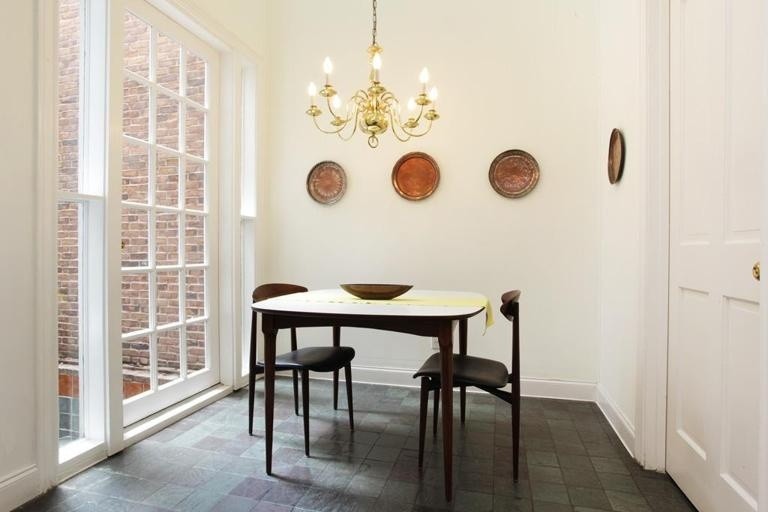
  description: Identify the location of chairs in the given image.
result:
[248,283,355,456]
[412,289,521,484]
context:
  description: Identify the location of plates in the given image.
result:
[340,283,412,300]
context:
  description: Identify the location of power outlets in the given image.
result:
[432,337,439,349]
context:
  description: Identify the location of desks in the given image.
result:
[250,290,489,504]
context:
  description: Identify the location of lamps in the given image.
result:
[305,44,440,148]
